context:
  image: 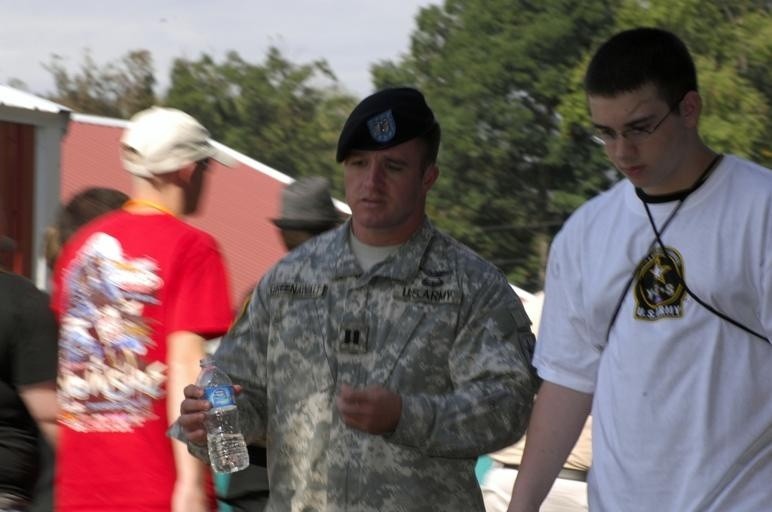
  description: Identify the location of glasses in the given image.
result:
[590,103,679,146]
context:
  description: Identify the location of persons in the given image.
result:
[505,29,772,511]
[50,106,237,511]
[169,86,543,512]
[2,187,133,511]
[266,176,351,253]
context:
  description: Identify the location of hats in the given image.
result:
[118,105,240,178]
[336,86,439,164]
[266,175,345,228]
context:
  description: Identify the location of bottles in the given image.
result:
[194,356,251,475]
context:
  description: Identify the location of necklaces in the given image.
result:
[122,200,176,217]
